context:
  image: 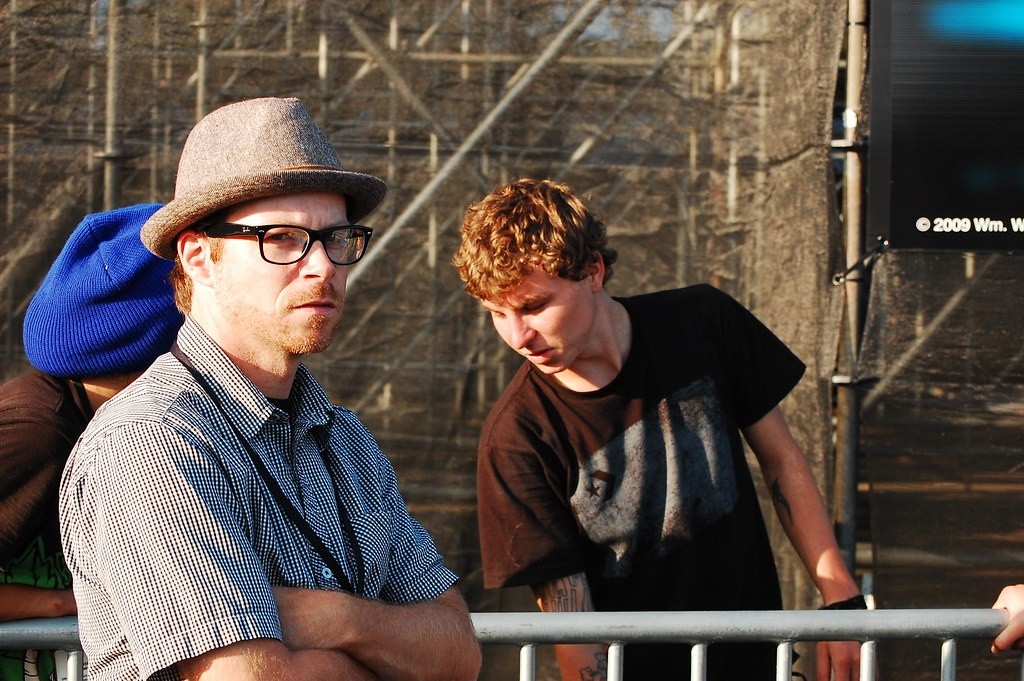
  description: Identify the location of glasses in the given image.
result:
[200,218,373,265]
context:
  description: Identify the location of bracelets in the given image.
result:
[818,594,867,610]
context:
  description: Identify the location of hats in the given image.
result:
[139,98,387,264]
[22,203,185,380]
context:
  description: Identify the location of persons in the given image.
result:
[0,203,185,681]
[450,177,868,681]
[58,95,483,681]
[990,584,1024,656]
